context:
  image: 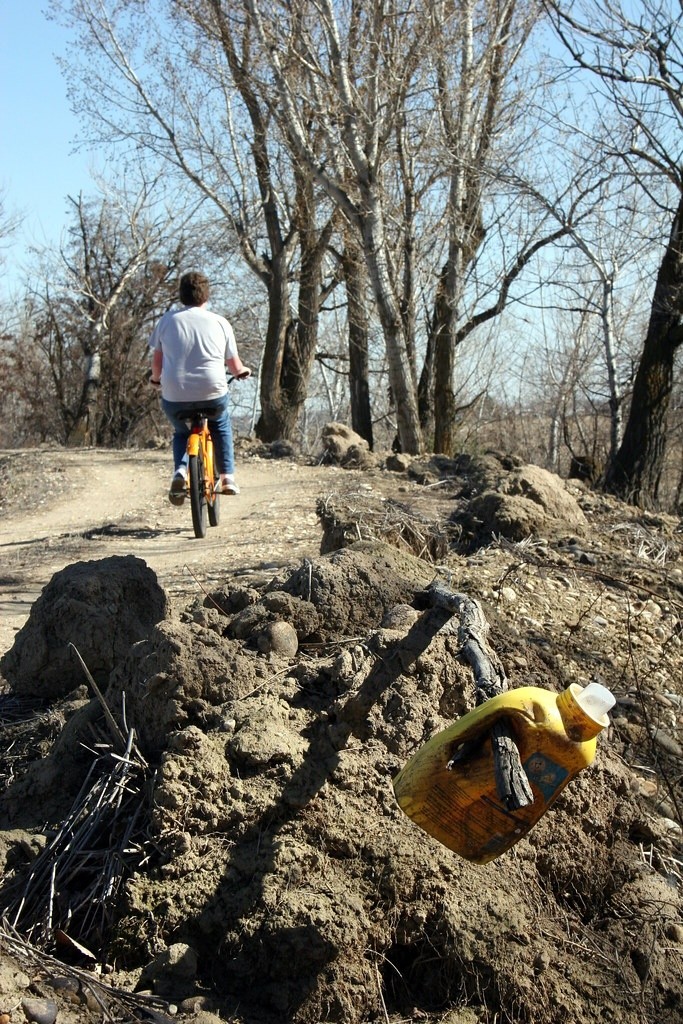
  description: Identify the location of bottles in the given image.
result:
[391,681,615,867]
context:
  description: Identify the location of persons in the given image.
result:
[149,272,252,506]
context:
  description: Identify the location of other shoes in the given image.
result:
[169,469,185,506]
[222,478,239,495]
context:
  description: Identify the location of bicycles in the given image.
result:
[147,371,252,541]
